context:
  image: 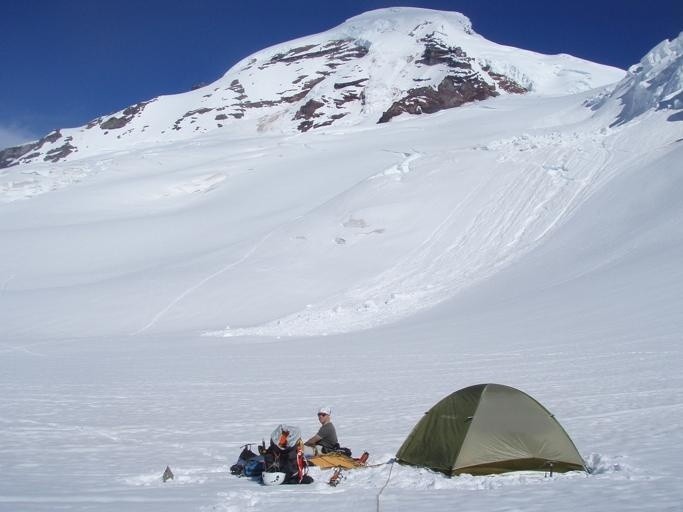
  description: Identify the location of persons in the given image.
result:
[304,406,338,453]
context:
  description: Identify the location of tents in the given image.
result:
[394,382,592,477]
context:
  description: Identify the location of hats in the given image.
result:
[319,408,331,415]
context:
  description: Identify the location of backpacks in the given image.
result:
[232,424,313,483]
